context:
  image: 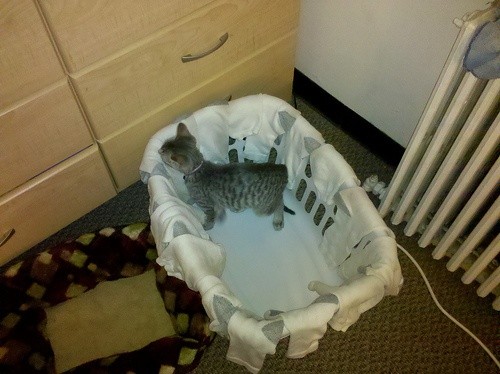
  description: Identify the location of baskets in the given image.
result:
[139,92,393,347]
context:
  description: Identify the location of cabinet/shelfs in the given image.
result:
[0,0,300,267]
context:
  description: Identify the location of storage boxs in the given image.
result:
[138,94,404,373]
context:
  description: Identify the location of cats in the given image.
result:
[158,122,295,232]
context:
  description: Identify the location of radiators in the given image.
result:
[363,0,500,311]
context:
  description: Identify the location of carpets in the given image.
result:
[0,222,217,374]
